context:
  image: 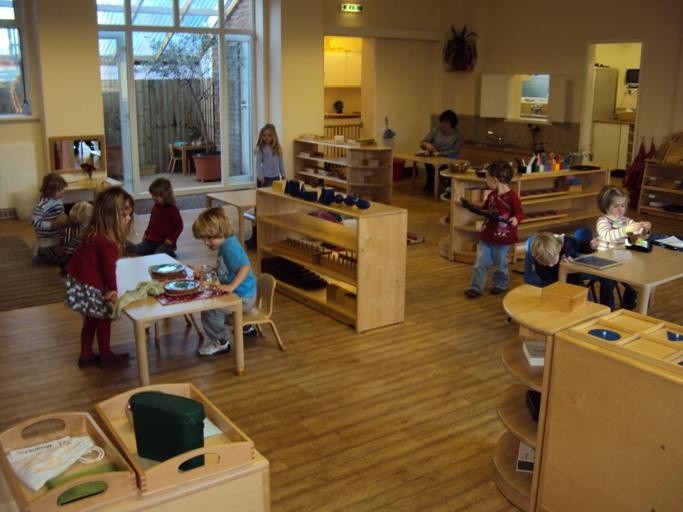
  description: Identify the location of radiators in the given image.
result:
[323,113,362,159]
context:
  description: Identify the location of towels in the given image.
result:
[114,281,164,322]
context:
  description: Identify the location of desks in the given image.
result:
[173,140,212,175]
[0,384,271,512]
[558,239,683,317]
[115,253,244,384]
[204,186,274,253]
[394,151,467,198]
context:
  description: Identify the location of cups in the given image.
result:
[200,274,216,305]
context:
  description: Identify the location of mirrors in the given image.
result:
[48,134,108,176]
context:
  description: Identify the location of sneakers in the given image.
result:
[463,288,482,298]
[196,338,231,357]
[77,352,99,367]
[231,324,258,337]
[99,351,132,367]
[488,286,507,294]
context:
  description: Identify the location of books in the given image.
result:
[304,166,334,176]
[346,138,378,148]
[297,150,325,159]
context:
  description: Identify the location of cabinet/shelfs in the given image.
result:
[294,138,393,208]
[492,283,683,512]
[440,166,611,264]
[256,185,409,331]
[637,158,683,222]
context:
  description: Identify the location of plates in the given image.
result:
[150,263,185,276]
[163,279,200,293]
[648,233,682,250]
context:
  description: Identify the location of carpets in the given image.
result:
[0,236,69,314]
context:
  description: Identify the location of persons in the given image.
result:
[595,185,651,313]
[126,178,184,259]
[530,232,599,285]
[29,173,70,268]
[63,187,135,368]
[192,207,258,357]
[419,110,463,192]
[65,201,93,255]
[464,159,525,298]
[255,123,287,188]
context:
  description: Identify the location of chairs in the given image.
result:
[222,273,287,355]
[167,143,190,174]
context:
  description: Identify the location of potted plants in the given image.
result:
[140,34,238,183]
[444,25,478,68]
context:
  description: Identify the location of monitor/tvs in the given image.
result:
[520,75,549,105]
[624,68,640,85]
